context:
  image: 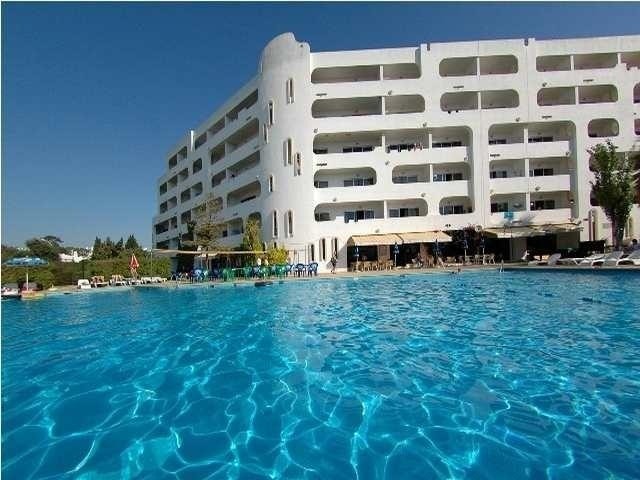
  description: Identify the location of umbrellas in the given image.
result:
[1,255,50,291]
[354,234,486,273]
[129,250,140,282]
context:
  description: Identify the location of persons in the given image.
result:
[619,238,640,260]
[331,251,339,275]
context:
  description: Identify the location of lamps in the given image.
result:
[531,200,534,210]
[568,198,576,206]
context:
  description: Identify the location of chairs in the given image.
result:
[529,250,640,267]
[1,282,38,297]
[76,274,169,288]
[174,263,318,279]
[409,254,496,265]
[351,260,393,272]
